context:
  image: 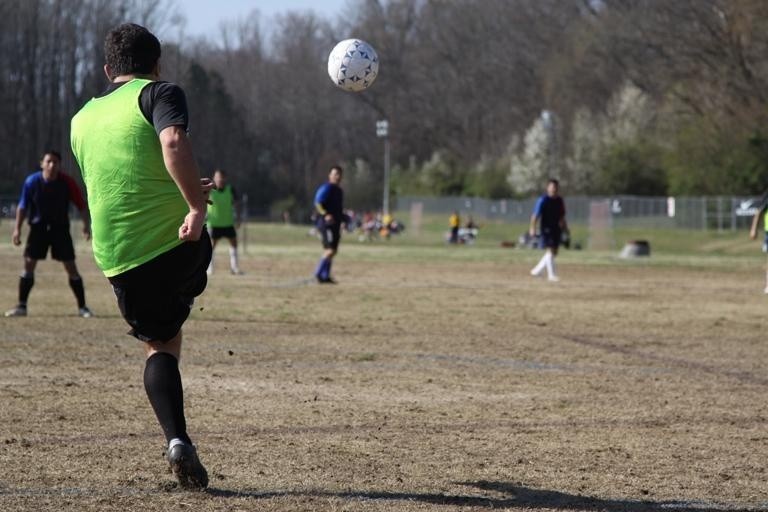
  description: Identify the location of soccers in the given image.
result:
[328,38,378,91]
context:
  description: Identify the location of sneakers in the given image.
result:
[4,308,26,316]
[79,308,92,318]
[167,438,207,491]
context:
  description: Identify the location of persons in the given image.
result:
[313,166,348,284]
[344,207,405,242]
[464,215,476,244]
[206,170,244,275]
[4,152,91,317]
[529,180,568,280]
[749,188,768,293]
[448,209,459,242]
[69,23,218,488]
